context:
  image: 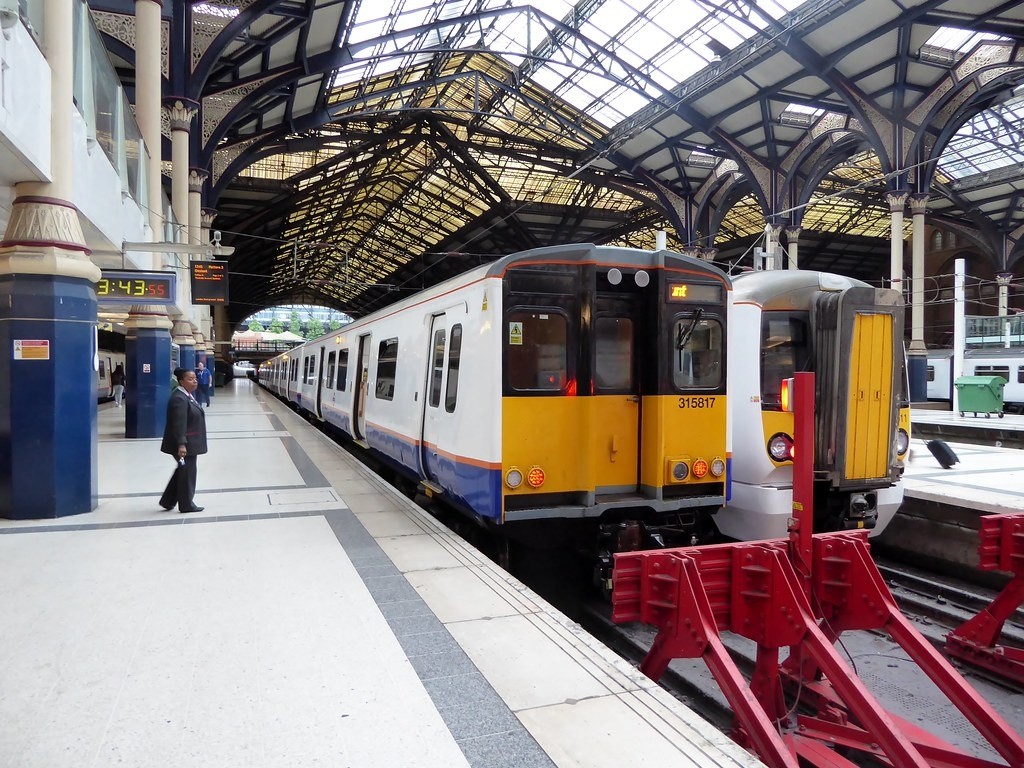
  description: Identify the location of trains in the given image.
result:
[256,243,735,603]
[709,267,914,542]
[97,347,125,399]
[926,346,1024,406]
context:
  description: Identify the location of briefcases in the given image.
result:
[910,423,960,469]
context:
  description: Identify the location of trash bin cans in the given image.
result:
[953,376,1009,418]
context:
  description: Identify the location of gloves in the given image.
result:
[209,384,212,388]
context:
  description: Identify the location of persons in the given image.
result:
[159,368,208,513]
[194,362,213,408]
[111,365,127,407]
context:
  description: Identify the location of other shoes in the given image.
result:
[181,507,204,513]
[207,403,210,406]
[115,402,118,407]
[160,501,174,511]
[118,405,122,408]
